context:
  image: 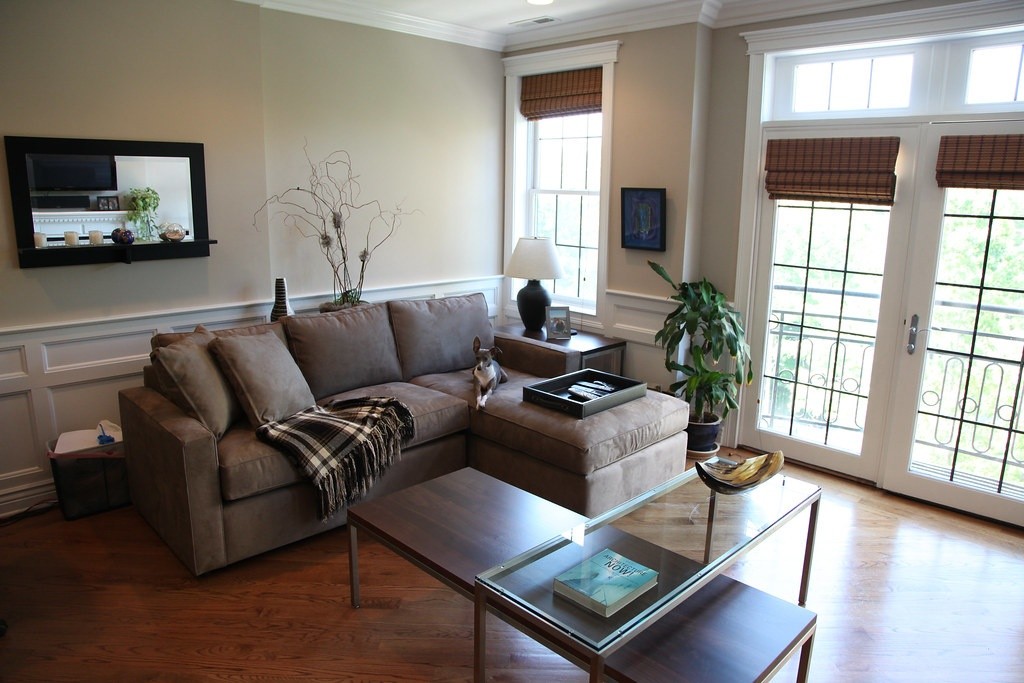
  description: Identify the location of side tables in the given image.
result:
[493,321,628,377]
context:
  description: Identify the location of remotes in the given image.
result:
[567,381,616,401]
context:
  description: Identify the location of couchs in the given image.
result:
[118,292,691,577]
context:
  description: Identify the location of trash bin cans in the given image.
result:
[44,437,133,522]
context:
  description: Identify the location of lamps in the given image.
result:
[504,236,565,332]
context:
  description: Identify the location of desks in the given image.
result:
[346,467,818,683]
[473,455,822,683]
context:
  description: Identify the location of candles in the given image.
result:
[89,230,104,244]
[34,232,49,247]
[64,232,80,246]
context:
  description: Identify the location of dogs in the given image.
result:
[472,336,508,411]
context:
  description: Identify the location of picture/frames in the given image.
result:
[621,186,666,252]
[545,306,571,340]
[97,195,120,211]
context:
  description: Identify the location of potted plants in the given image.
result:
[646,260,754,451]
[124,187,161,243]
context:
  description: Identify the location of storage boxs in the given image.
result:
[45,437,131,522]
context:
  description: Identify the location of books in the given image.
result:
[554,548,660,618]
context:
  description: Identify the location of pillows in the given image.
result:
[386,292,495,382]
[149,323,237,442]
[207,329,316,428]
[151,321,288,349]
[278,302,403,401]
